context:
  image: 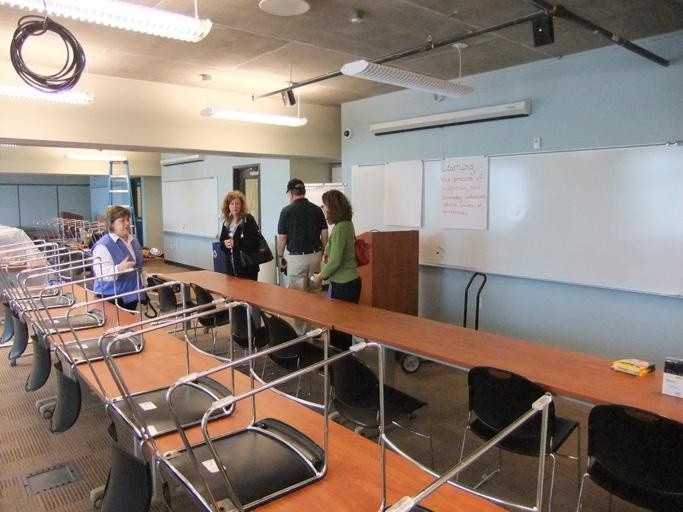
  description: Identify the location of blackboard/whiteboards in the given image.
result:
[161,176,219,239]
[349,140,683,298]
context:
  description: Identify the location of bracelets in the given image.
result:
[278,256,283,257]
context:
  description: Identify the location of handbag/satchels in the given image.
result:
[353,237,372,267]
[236,213,274,270]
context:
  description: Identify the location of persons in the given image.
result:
[313,191,362,350]
[278,179,328,337]
[91,206,144,315]
[220,192,260,281]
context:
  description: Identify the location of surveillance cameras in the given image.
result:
[343,128,352,139]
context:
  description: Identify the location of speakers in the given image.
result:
[281,90,296,107]
[532,14,554,46]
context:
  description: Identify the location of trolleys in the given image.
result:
[395,271,489,371]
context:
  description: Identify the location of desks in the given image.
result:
[153,270,682,422]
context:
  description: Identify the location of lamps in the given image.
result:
[338,8,475,101]
[0,53,94,105]
[278,9,557,110]
[1,0,214,44]
[198,72,308,127]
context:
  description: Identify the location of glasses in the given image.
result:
[321,205,326,209]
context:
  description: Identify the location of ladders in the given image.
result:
[108,158,138,238]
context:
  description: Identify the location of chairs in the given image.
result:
[0,218,186,434]
[98,281,583,512]
[576,404,682,512]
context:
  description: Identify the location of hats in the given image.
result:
[285,178,306,194]
